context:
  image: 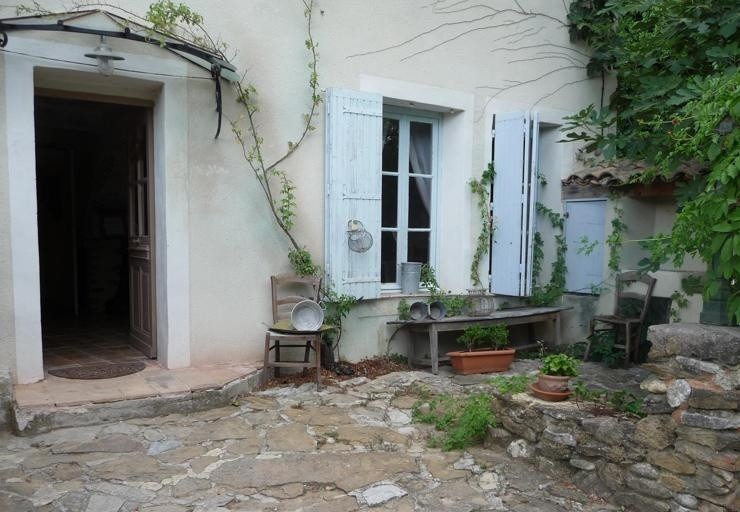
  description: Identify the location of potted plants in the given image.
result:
[529,354,580,402]
[445,321,516,374]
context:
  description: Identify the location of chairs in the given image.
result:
[262,276,323,392]
[582,271,657,371]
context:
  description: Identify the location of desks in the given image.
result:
[386,303,574,373]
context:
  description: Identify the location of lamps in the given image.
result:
[84,33,126,76]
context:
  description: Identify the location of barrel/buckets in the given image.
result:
[401,262,422,294]
[291,300,326,331]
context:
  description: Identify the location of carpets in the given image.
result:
[48,362,146,380]
